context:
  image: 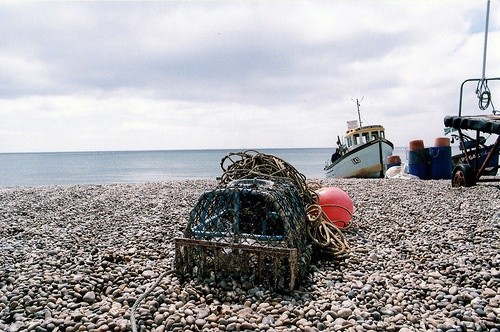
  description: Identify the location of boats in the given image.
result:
[324,96,394,179]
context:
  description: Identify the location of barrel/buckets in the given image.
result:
[409,146,451,180]
[386,163,400,170]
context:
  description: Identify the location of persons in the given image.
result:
[331,148,341,164]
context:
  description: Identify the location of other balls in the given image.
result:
[313,187,353,227]
